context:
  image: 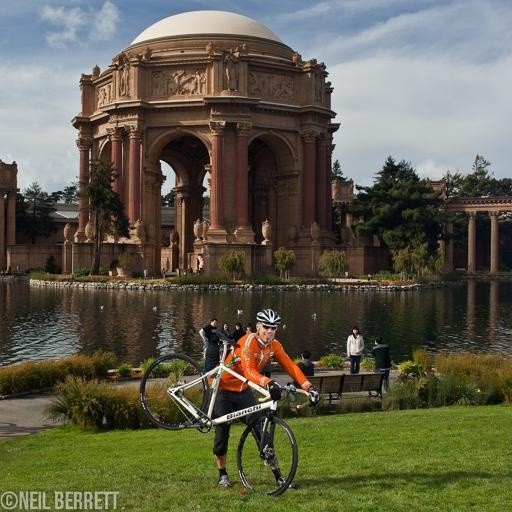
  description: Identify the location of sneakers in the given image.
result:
[278,477,295,487]
[217,474,231,488]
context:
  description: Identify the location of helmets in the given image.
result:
[256,309,282,328]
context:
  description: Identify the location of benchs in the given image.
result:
[299,373,385,404]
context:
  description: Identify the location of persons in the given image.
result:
[198,318,252,374]
[286,350,315,388]
[210,306,320,489]
[370,336,393,393]
[346,327,365,373]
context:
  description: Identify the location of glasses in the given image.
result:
[261,323,276,330]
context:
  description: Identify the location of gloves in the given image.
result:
[307,386,320,407]
[267,380,282,401]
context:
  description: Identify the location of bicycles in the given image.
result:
[139,329,313,498]
